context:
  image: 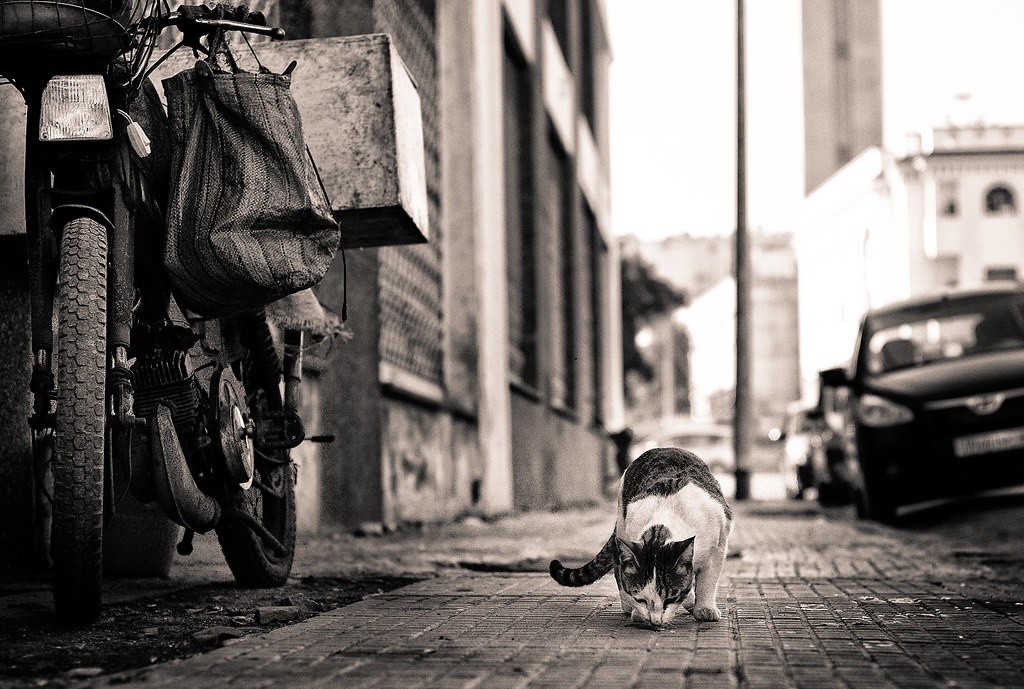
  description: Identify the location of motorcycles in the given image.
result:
[0,3,338,629]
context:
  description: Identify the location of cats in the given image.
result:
[549,446,733,628]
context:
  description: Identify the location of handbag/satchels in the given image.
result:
[162,26,340,318]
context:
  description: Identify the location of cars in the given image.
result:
[816,278,1024,521]
[802,365,859,506]
[768,398,819,499]
